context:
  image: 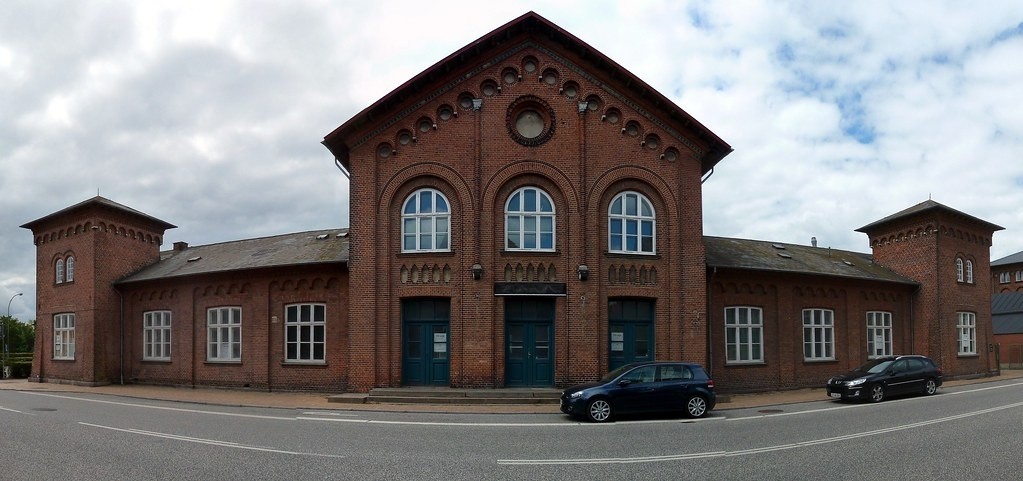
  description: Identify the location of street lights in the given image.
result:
[7,292,23,358]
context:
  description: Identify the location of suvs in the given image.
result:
[825,354,942,403]
[558,361,716,423]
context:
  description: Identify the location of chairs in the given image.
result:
[644,367,654,382]
